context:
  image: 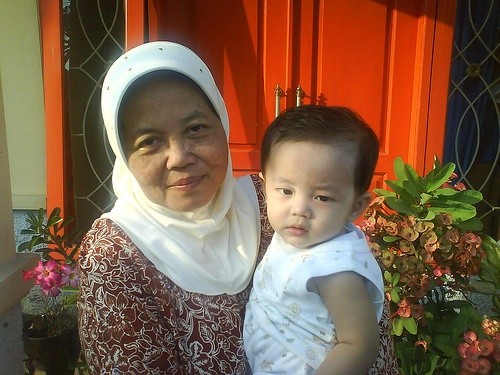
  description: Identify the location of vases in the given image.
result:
[23,314,80,375]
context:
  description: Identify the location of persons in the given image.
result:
[77,41,398,375]
[244,105,385,375]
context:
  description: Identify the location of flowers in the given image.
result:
[21,259,78,332]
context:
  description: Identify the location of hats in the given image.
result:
[102,41,261,297]
